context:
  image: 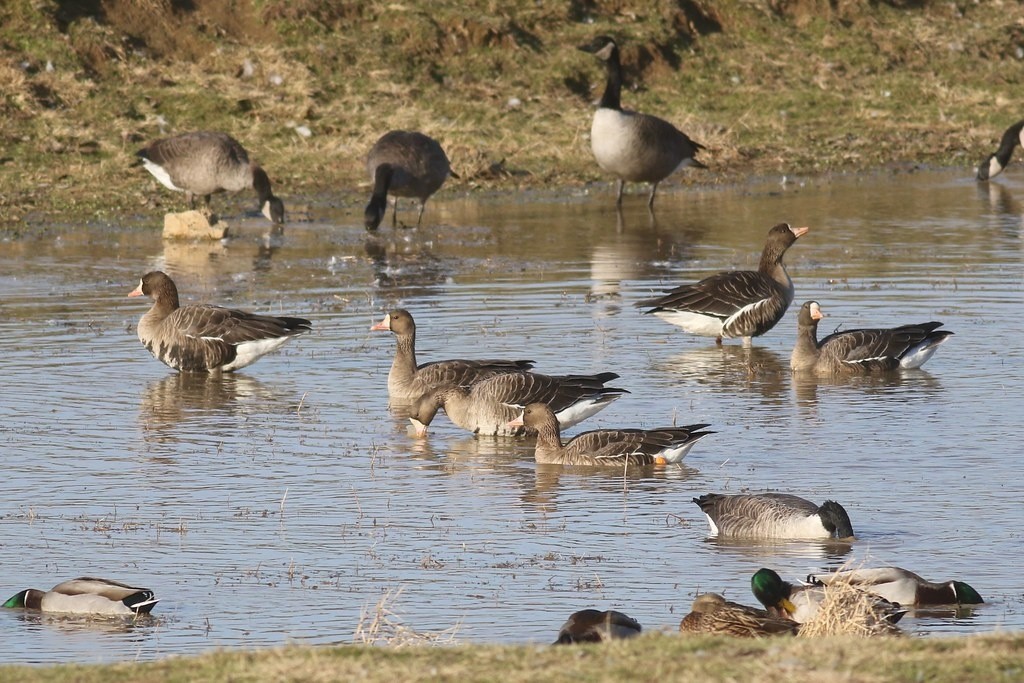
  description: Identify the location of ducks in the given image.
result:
[1,576,163,615]
[679,566,910,641]
[797,565,984,607]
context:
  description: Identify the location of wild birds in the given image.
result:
[505,401,719,467]
[408,370,632,438]
[974,120,1024,181]
[789,300,956,375]
[575,35,712,209]
[630,222,809,350]
[690,492,860,542]
[362,129,461,232]
[369,309,538,412]
[126,130,284,228]
[549,609,641,645]
[127,271,315,374]
